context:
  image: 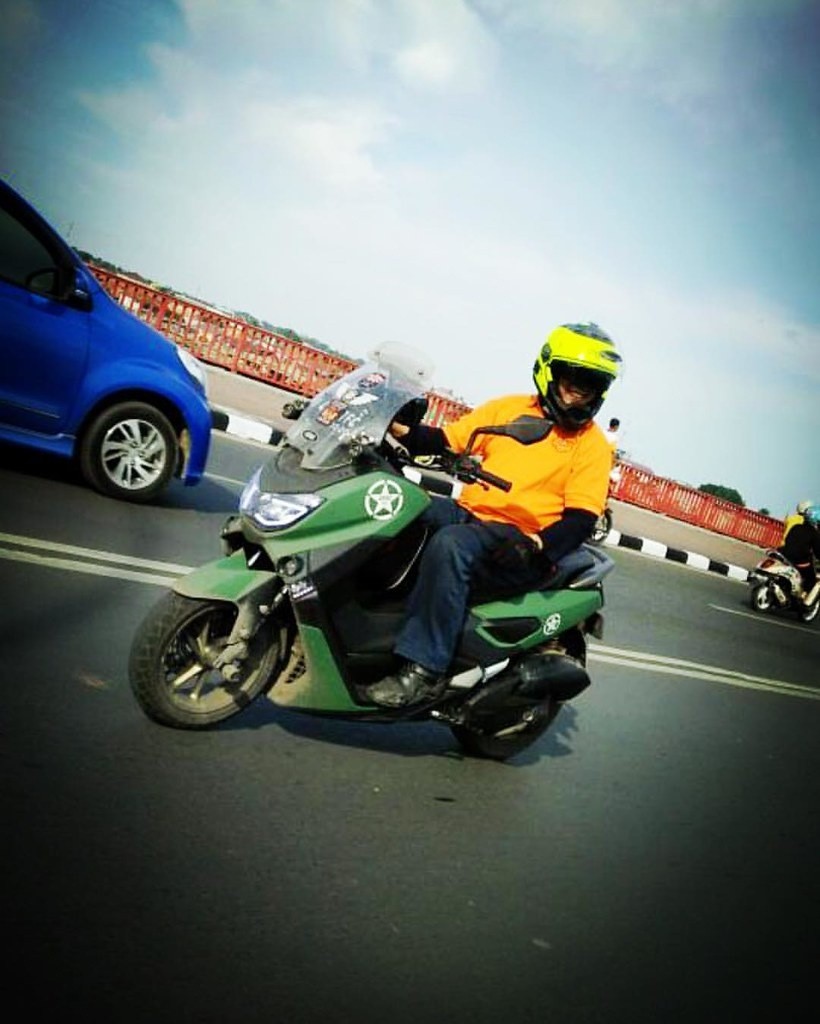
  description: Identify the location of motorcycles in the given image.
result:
[745,548,820,625]
[128,355,618,761]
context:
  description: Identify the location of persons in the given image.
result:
[779,500,820,595]
[365,321,623,709]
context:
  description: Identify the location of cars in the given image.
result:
[0,183,212,502]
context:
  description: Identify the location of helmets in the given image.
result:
[805,506,820,522]
[533,322,623,432]
[797,502,812,513]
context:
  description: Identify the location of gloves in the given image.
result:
[490,530,540,567]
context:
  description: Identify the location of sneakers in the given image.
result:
[365,661,446,708]
